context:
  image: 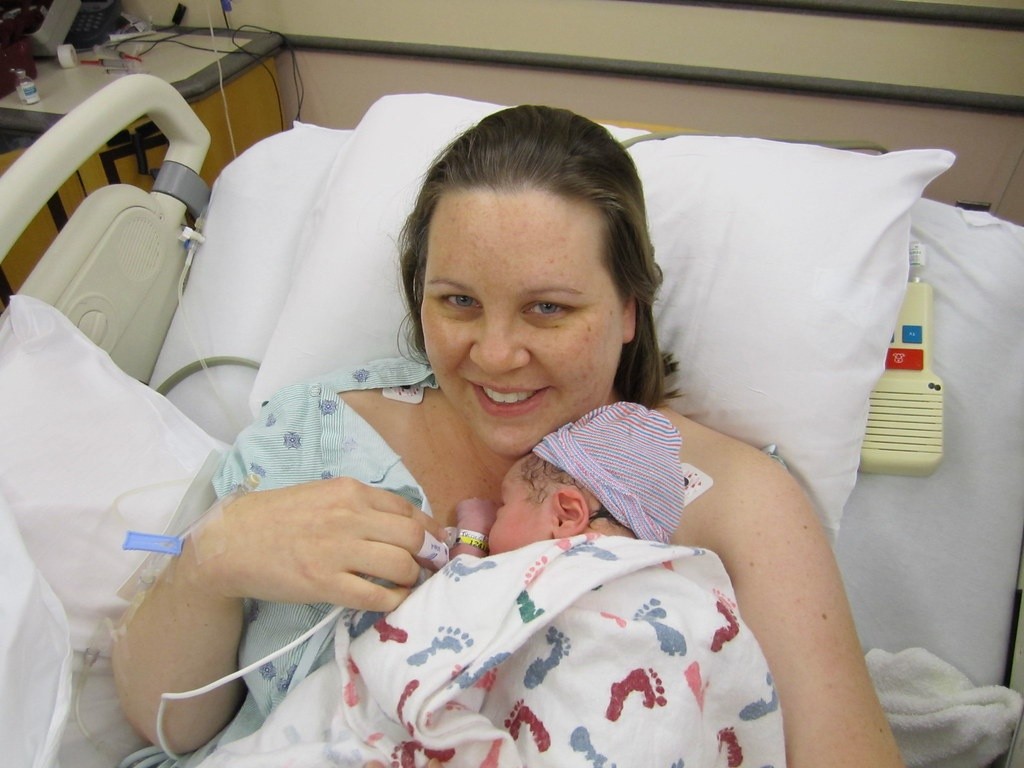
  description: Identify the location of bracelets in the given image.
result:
[458,530,488,553]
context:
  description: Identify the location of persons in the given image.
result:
[112,105,903,768]
[446,401,685,767]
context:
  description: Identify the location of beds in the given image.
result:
[0,72,1024,768]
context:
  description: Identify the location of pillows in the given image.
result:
[0,295,234,768]
[248,94,957,540]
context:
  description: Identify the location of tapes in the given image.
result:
[57,44,77,69]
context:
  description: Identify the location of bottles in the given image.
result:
[16,70,39,104]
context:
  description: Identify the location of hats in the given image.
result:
[533,400,684,541]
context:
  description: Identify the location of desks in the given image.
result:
[0,25,289,315]
